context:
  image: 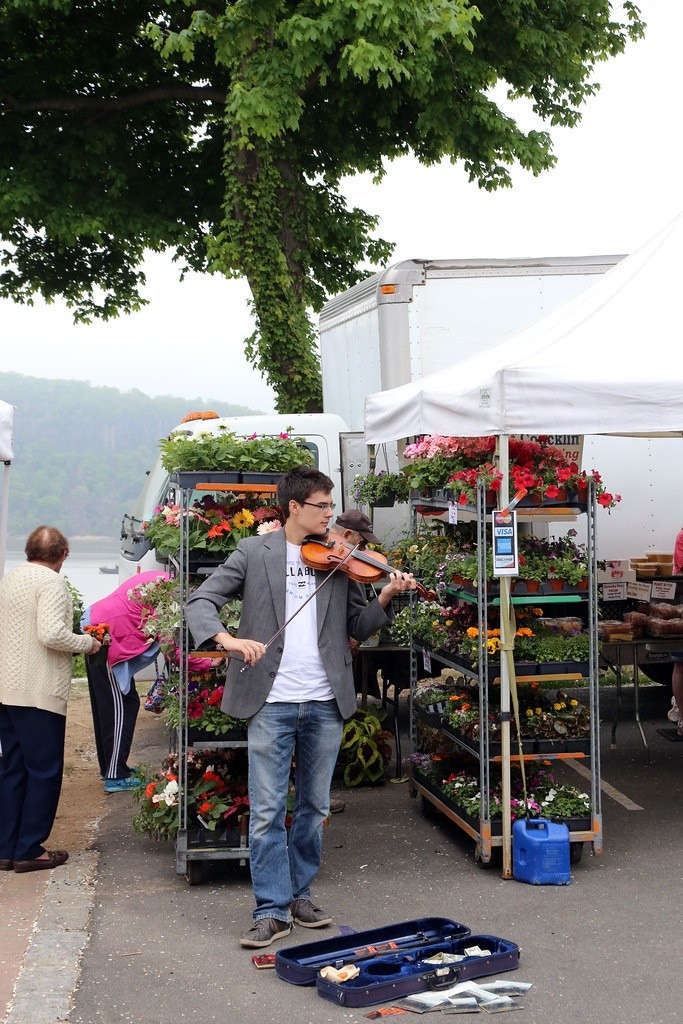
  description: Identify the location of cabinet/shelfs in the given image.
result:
[412,437,603,864]
[152,472,294,883]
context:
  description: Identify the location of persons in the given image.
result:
[330,509,382,811]
[668,528,683,737]
[79,571,224,793]
[0,525,102,873]
[182,466,416,949]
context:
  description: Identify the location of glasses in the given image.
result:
[298,501,337,512]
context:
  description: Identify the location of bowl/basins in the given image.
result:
[630,552,674,577]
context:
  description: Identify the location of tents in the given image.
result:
[0,398,18,582]
[364,208,683,881]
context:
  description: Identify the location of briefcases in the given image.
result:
[275,917,520,1008]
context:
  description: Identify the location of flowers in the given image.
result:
[130,749,296,839]
[397,524,589,590]
[240,431,314,470]
[387,600,605,661]
[413,684,589,737]
[126,575,242,654]
[141,493,285,560]
[65,576,111,647]
[352,436,621,505]
[154,675,248,735]
[401,753,590,819]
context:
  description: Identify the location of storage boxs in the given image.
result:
[597,560,636,583]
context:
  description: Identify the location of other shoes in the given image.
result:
[1,858,14,871]
[104,778,146,795]
[13,850,69,873]
[668,695,683,736]
[100,767,142,781]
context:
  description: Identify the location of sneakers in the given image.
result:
[291,899,332,931]
[239,917,290,947]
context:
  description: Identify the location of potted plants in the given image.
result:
[157,430,239,488]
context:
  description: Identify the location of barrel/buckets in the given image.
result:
[513,817,571,886]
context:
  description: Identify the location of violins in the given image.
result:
[300,528,437,602]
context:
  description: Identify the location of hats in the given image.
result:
[335,509,382,545]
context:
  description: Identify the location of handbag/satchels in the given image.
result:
[144,678,168,713]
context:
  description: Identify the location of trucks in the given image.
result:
[123,253,683,686]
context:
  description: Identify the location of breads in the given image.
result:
[600,602,683,638]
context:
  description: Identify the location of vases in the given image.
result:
[155,546,233,570]
[240,472,284,484]
[181,725,246,748]
[181,812,240,848]
[73,630,84,656]
[411,771,591,835]
[368,485,587,508]
[416,709,591,755]
[452,575,588,596]
[413,637,589,681]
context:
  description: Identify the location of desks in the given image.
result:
[359,643,409,710]
[601,634,683,768]
